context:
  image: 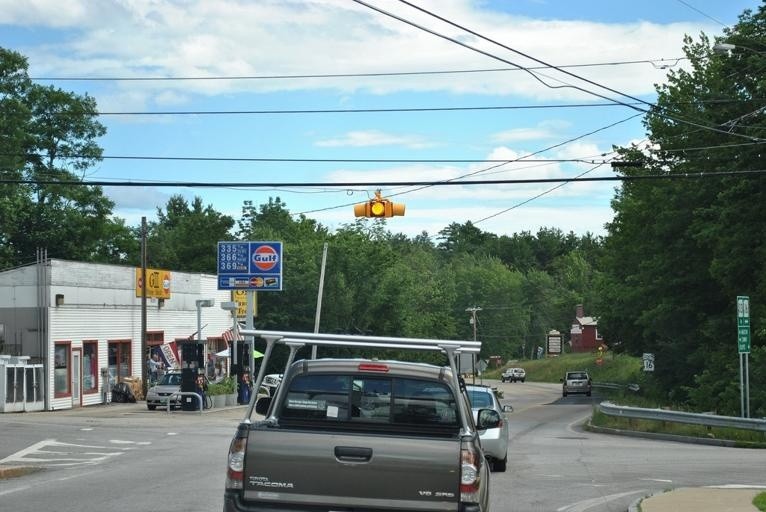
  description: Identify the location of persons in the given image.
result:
[148,352,163,385]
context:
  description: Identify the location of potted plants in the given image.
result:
[204,375,240,409]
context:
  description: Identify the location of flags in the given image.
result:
[222,325,244,349]
[150,340,180,370]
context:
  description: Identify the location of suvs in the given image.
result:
[501,368,525,383]
[560,371,591,397]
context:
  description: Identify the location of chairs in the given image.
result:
[394,392,440,422]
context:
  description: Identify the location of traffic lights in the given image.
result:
[354,192,407,219]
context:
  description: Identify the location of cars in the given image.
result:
[146,373,183,410]
[421,386,512,473]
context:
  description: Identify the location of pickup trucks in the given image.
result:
[223,330,490,511]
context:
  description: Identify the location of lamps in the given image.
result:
[56,294,64,306]
[158,299,165,308]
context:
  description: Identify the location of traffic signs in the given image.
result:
[737,297,751,353]
[643,353,655,371]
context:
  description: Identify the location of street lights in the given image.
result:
[465,307,484,340]
